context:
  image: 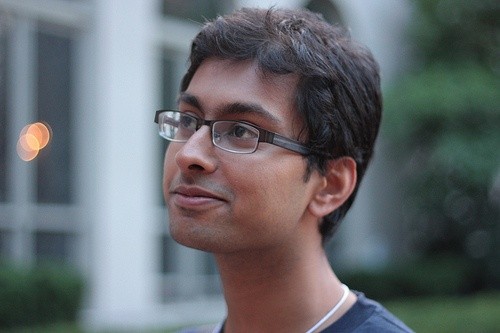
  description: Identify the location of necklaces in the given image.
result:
[305,283,350,333]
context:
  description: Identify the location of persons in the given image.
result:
[154,6,415,333]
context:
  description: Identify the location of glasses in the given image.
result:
[155,110,333,158]
